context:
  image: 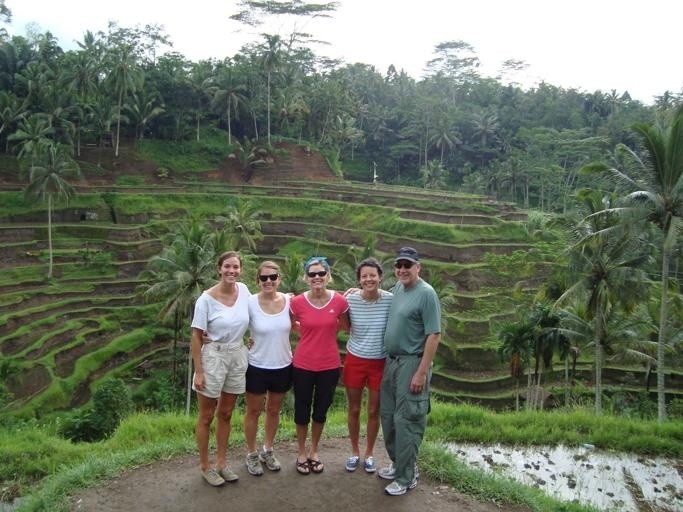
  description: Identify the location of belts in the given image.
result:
[389,352,423,359]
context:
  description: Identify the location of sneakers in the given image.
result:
[259,449,281,470]
[378,462,420,480]
[363,455,376,472]
[245,452,264,475]
[346,454,361,471]
[214,465,239,481]
[385,479,417,495]
[199,467,225,486]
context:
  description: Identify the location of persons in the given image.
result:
[198,260,295,476]
[246,255,350,476]
[189,251,253,486]
[348,245,443,496]
[341,256,393,473]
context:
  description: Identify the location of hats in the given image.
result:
[396,247,419,263]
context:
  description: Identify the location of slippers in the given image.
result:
[297,457,310,473]
[308,456,324,473]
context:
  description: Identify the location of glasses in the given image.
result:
[259,274,278,281]
[394,262,414,268]
[307,270,326,277]
